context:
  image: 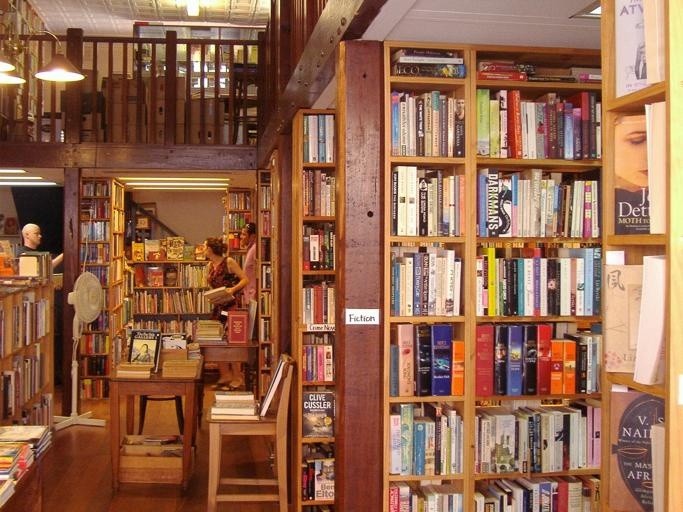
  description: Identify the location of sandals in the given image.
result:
[211,381,246,392]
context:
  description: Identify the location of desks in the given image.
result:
[109,375,198,494]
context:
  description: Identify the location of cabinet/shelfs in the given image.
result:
[462,43,602,510]
[76,167,272,406]
[0,280,51,432]
[379,45,472,512]
[599,0,680,512]
[296,112,341,510]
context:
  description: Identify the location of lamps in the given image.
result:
[1,3,87,89]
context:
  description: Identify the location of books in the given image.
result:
[0,294,51,507]
[387,47,603,512]
[208,362,287,424]
[78,182,258,401]
[262,184,275,394]
[302,117,336,512]
[604,1,667,511]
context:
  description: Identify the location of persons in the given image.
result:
[19,221,63,269]
[133,345,151,362]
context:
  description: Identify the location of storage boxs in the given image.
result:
[58,67,247,144]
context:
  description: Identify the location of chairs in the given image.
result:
[209,357,293,512]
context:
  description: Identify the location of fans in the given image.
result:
[53,273,105,436]
